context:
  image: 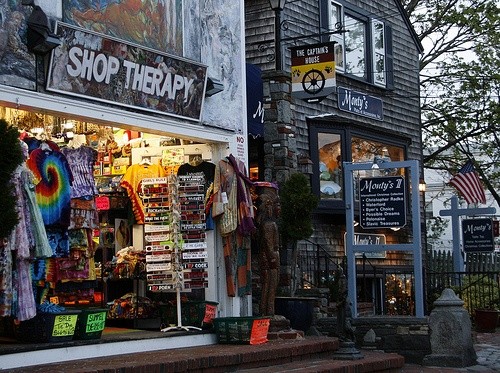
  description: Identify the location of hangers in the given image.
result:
[139,149,154,166]
[67,133,82,149]
[189,148,204,165]
[37,132,52,151]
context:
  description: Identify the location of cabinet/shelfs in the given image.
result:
[101,262,160,329]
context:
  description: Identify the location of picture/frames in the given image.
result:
[45,20,209,123]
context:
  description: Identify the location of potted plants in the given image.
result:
[475,299,500,333]
[274,173,320,332]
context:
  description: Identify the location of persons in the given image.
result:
[259,198,280,317]
[334,265,350,343]
[116,220,129,249]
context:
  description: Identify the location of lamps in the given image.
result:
[28,28,61,55]
[206,78,225,97]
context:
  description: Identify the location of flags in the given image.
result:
[448,160,486,205]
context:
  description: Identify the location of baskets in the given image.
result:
[214,316,272,344]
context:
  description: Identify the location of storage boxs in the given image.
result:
[158,301,220,329]
[36,310,82,344]
[68,309,111,340]
[212,316,272,345]
